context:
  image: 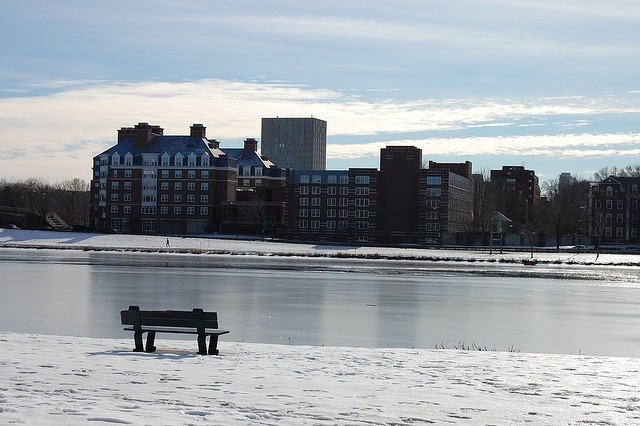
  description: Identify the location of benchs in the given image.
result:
[119,304,231,355]
